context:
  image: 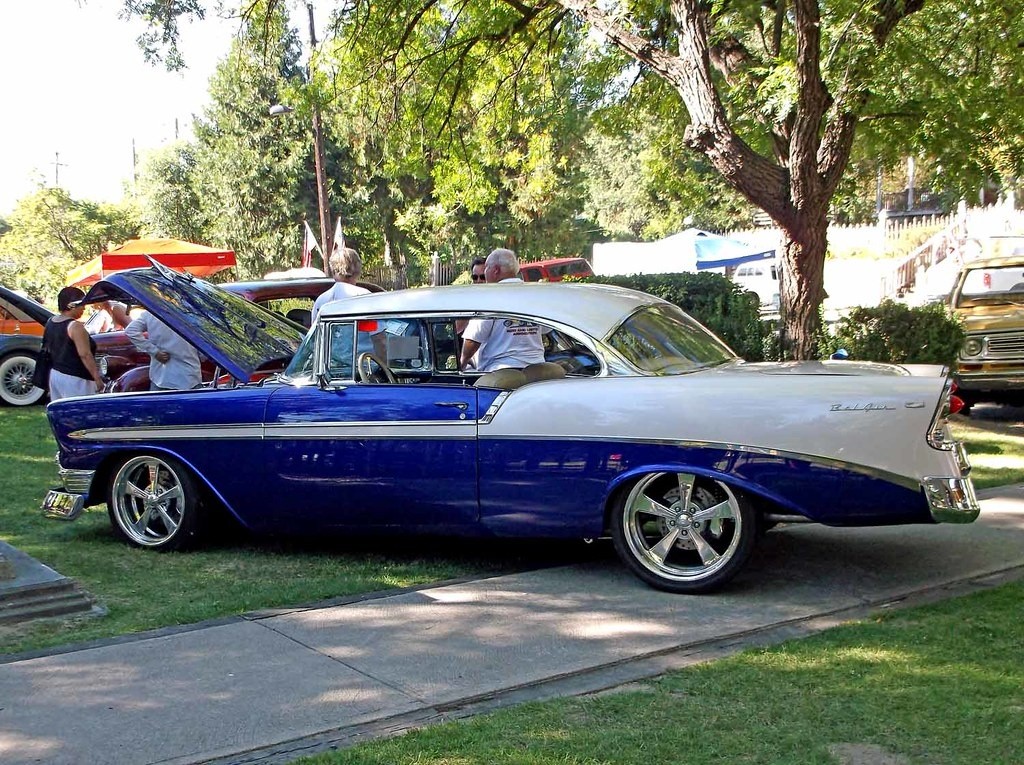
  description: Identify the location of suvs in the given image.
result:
[519,257,593,281]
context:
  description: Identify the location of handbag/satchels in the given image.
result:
[32,350,51,389]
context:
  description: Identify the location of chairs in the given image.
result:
[474,362,566,389]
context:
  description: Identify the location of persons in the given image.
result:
[456,258,486,335]
[124,310,209,390]
[458,248,553,372]
[310,248,387,372]
[93,299,129,331]
[43,286,105,401]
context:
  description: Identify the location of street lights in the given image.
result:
[269,104,332,278]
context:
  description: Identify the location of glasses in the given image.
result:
[471,274,485,280]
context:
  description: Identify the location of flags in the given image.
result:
[332,218,346,253]
[303,222,316,267]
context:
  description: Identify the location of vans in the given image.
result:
[734,258,780,306]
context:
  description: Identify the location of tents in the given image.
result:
[67,238,237,289]
[593,228,776,275]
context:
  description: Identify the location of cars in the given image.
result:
[42,253,979,593]
[90,277,390,395]
[0,285,58,407]
[940,257,1024,415]
